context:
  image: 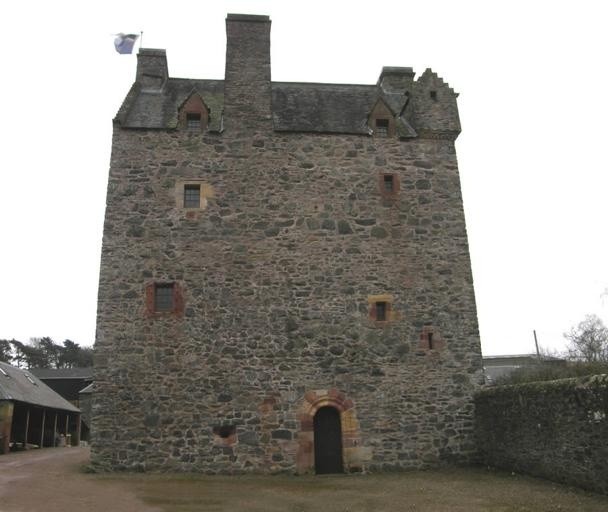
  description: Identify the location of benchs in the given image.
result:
[114,32,141,55]
